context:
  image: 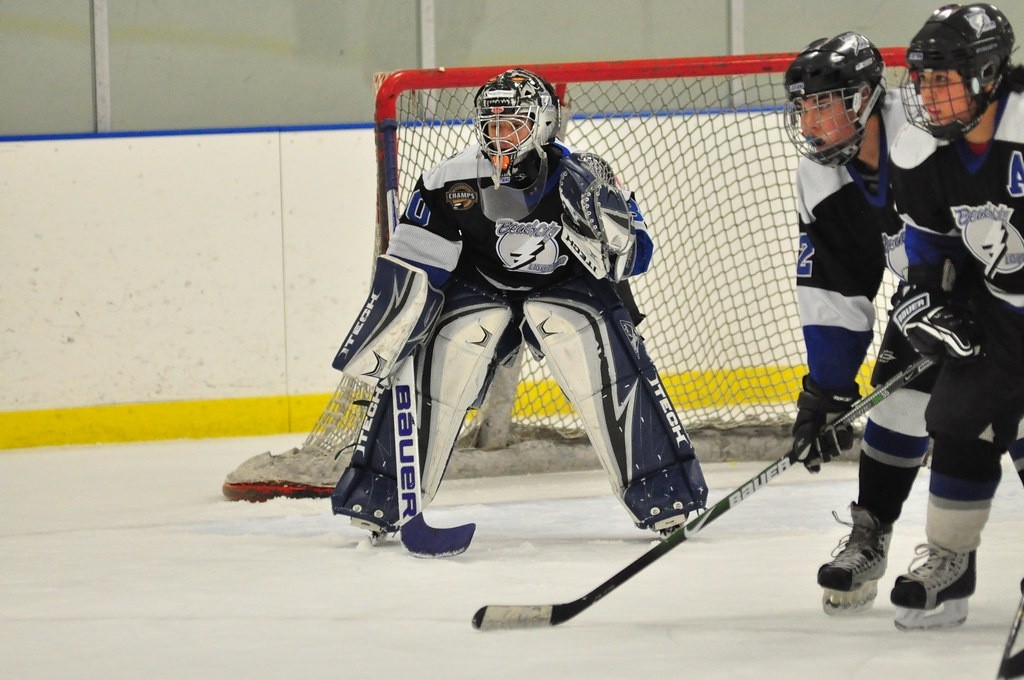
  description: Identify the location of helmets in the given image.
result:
[474,66,563,173]
[783,31,888,172]
[899,3,1017,143]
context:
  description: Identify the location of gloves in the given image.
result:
[892,280,990,366]
[793,373,855,474]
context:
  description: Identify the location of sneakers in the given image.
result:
[889,544,977,632]
[817,503,893,614]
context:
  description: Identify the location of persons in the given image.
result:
[779,31,1023,616]
[327,66,709,549]
[879,3,1024,680]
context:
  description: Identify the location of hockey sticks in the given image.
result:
[471,360,933,635]
[377,119,477,559]
[994,594,1024,680]
